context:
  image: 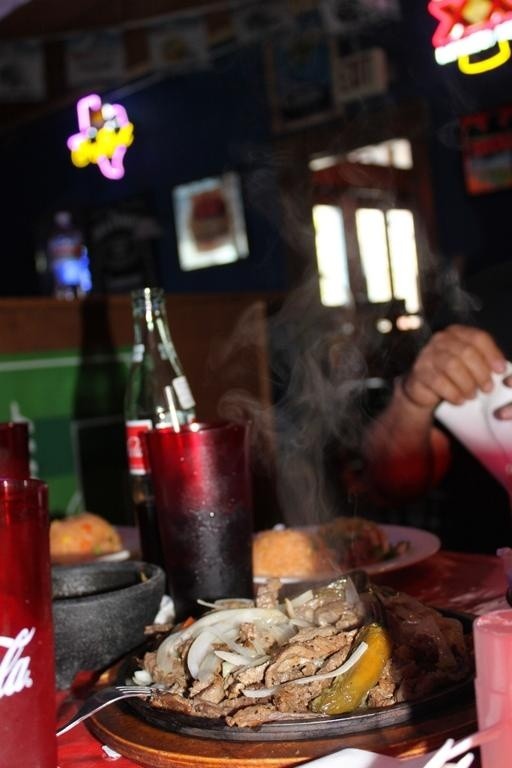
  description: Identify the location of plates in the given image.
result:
[93,526,141,562]
[253,523,441,585]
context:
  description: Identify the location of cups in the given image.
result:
[144,422,263,626]
[472,609,512,768]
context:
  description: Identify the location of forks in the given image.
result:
[56,685,160,740]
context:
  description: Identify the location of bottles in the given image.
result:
[47,211,93,303]
[0,421,58,768]
[123,287,209,563]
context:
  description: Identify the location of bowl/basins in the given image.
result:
[51,562,166,690]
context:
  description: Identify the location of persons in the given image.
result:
[353,287,512,556]
